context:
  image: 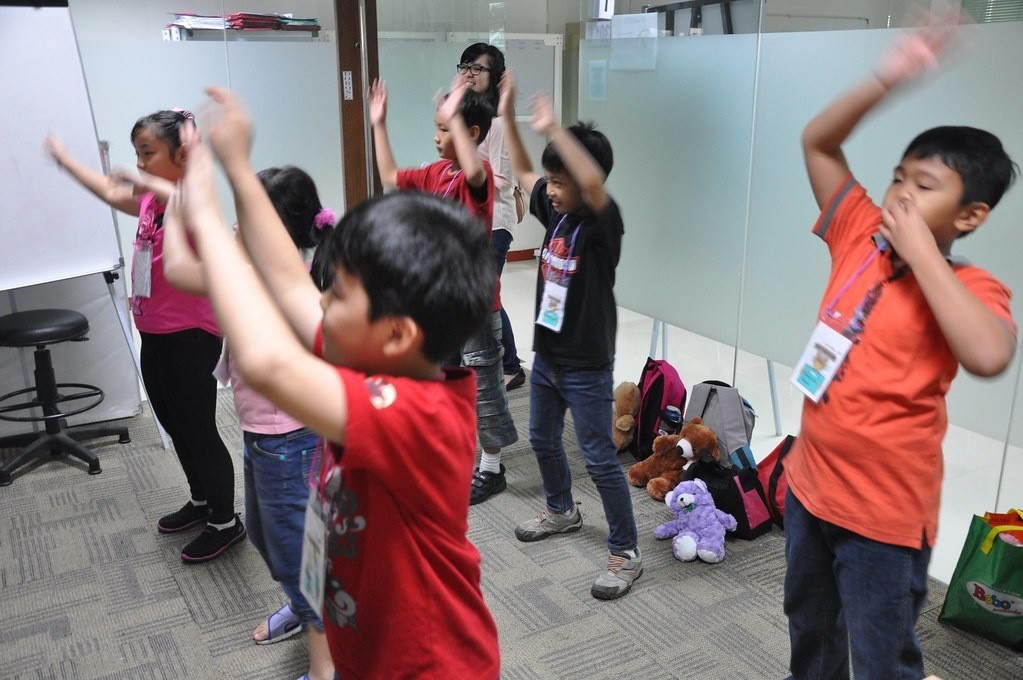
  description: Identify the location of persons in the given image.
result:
[783,0,1022,680]
[43,42,648,680]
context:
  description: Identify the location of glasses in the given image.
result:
[457,64,489,75]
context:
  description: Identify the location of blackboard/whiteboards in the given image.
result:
[0,0,124,292]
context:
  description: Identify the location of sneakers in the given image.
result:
[515,500,583,542]
[158,501,207,533]
[468,463,507,505]
[181,512,246,563]
[591,543,645,602]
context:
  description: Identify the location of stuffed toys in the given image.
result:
[607,380,738,564]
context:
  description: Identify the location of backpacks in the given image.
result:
[629,356,687,462]
[684,381,760,471]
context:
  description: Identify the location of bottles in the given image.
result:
[657,405,681,436]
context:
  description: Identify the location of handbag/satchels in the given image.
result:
[938,508,1023,654]
[684,448,773,541]
[755,435,797,531]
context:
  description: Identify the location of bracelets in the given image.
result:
[868,64,890,92]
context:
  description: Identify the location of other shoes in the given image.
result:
[505,369,526,391]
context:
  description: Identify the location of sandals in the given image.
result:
[254,602,304,645]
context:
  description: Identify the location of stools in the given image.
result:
[0,309,131,488]
